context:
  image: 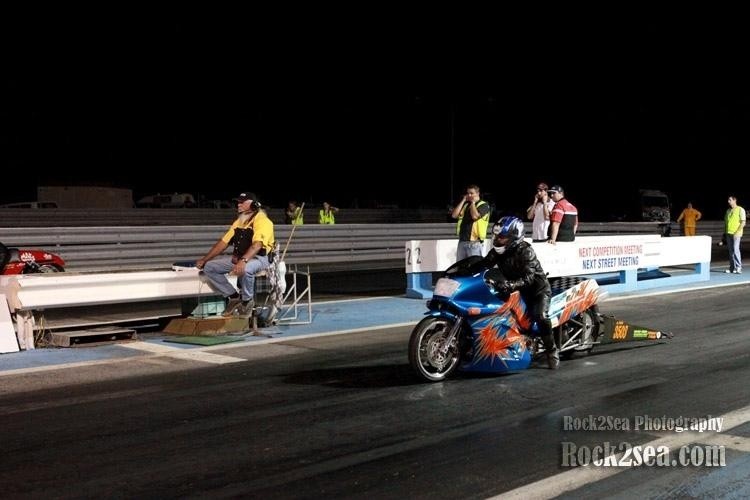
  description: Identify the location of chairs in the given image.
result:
[224,239,285,341]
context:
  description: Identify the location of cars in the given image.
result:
[0,243,66,276]
[137,193,239,207]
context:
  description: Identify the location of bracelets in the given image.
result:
[542,201,547,204]
[471,202,475,205]
[240,257,248,263]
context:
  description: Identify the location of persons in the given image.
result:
[657,202,673,237]
[527,182,556,242]
[452,185,490,263]
[184,197,193,207]
[484,215,559,369]
[198,191,276,320]
[317,201,340,224]
[722,196,747,274]
[287,201,304,224]
[545,185,579,244]
[677,202,703,236]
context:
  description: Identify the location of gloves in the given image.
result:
[496,279,516,294]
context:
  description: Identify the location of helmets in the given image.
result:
[492,215,525,256]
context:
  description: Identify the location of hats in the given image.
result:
[546,184,564,193]
[231,191,257,204]
[535,182,549,191]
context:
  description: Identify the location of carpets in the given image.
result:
[163,334,245,346]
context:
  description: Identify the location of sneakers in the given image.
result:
[238,300,255,319]
[220,295,243,317]
[548,348,561,369]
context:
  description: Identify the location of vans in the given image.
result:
[638,189,672,222]
[4,202,59,210]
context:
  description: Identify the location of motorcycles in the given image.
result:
[408,256,602,383]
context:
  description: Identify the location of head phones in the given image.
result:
[250,199,261,211]
